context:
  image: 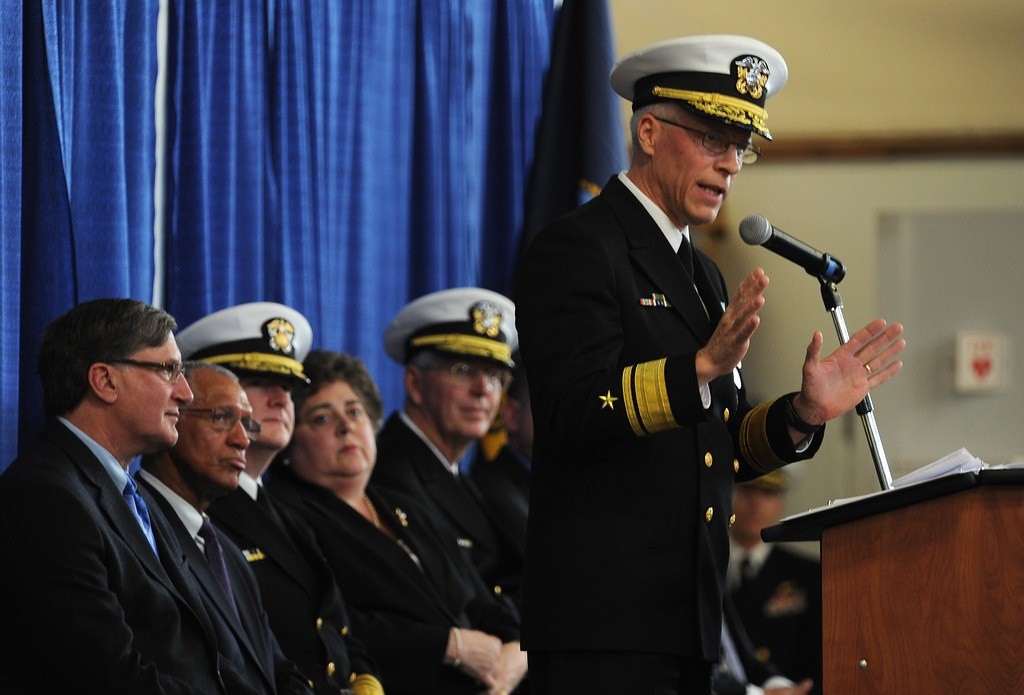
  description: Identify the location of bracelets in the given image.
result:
[452,627,464,667]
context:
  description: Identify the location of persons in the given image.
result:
[266,346,566,695]
[517,36,911,694]
[359,288,572,695]
[0,299,230,695]
[130,359,309,695]
[718,467,823,695]
[174,299,386,695]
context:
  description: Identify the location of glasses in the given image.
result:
[178,407,261,442]
[416,358,514,393]
[107,358,186,383]
[654,117,763,165]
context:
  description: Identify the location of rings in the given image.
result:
[864,363,874,375]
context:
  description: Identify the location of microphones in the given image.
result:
[740,215,847,284]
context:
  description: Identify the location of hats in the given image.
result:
[608,35,788,142]
[383,287,520,369]
[172,301,314,387]
[742,464,797,493]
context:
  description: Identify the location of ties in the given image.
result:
[197,517,241,625]
[677,234,694,284]
[740,554,751,589]
[256,484,296,550]
[122,474,160,563]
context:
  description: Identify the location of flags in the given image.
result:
[517,1,628,286]
